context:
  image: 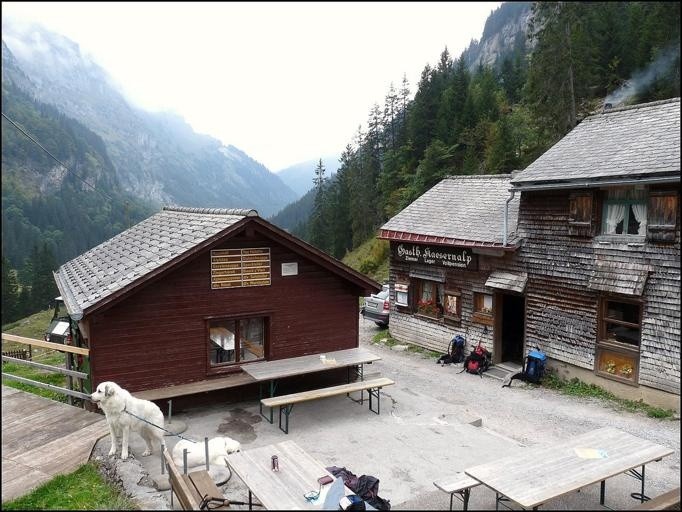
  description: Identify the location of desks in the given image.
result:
[464,426,674,511]
[225,440,380,511]
[239,348,382,424]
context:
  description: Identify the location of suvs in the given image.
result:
[356,284,390,330]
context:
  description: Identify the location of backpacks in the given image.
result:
[449,334,465,364]
[464,345,491,376]
[524,345,547,385]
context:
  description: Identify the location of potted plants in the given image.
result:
[432,307,441,319]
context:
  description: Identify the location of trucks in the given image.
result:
[44,295,73,354]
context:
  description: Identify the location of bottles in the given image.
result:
[270,454,280,472]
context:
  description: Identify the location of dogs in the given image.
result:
[89,381,167,461]
[171,436,241,468]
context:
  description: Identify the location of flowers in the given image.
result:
[416,298,433,310]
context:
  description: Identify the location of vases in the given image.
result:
[418,310,428,316]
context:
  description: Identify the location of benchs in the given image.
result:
[240,337,265,360]
[434,471,482,511]
[626,486,681,511]
[163,449,232,512]
[260,377,395,434]
